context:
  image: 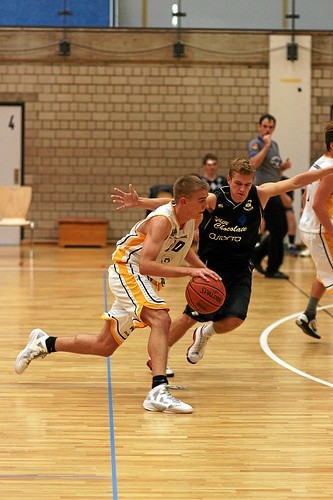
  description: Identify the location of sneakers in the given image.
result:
[14,329,50,374]
[295,313,321,338]
[186,325,212,364]
[147,357,175,377]
[142,383,193,413]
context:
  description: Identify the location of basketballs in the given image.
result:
[186,275,226,314]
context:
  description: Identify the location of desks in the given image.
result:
[56,217,110,248]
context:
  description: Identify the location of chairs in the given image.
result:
[0,186,37,269]
[147,185,176,216]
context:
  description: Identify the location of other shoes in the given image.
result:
[249,251,266,274]
[289,244,296,254]
[265,268,289,278]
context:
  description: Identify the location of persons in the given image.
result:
[111,157,333,378]
[15,175,222,414]
[298,125,333,339]
[249,114,289,279]
[279,177,297,257]
[200,153,230,193]
